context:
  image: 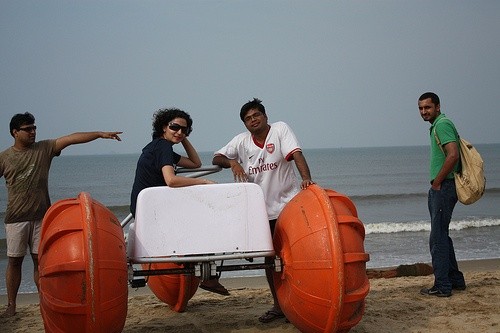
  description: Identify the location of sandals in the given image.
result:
[258,310,285,323]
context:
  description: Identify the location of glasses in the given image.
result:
[20,126,37,133]
[168,122,193,135]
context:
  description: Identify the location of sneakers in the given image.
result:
[451,283,466,290]
[420,285,451,297]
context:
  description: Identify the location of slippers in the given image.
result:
[198,283,230,296]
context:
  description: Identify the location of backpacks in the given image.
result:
[433,117,487,206]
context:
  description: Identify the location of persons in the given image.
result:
[0,113,123,317]
[129,109,231,296]
[418,94,467,298]
[212,98,318,324]
